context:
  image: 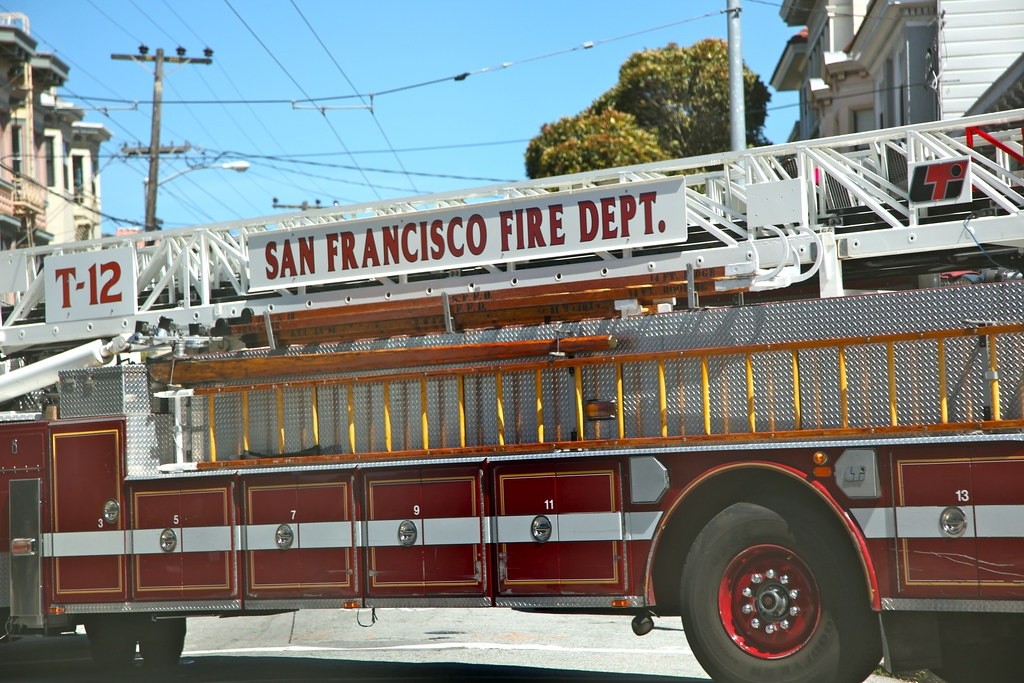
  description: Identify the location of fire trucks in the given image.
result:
[0,109,1024,683]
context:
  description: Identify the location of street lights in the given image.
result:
[142,161,249,245]
